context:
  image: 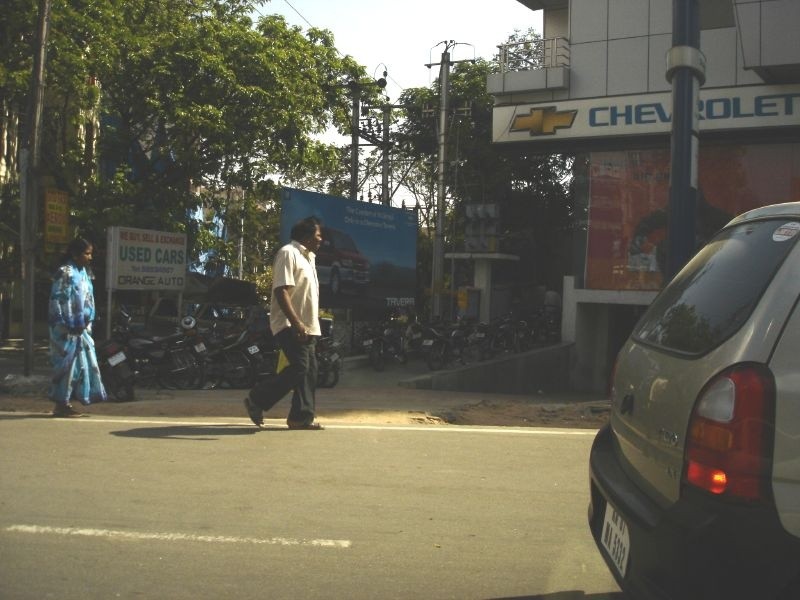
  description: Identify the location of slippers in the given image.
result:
[244,398,265,428]
[289,423,324,430]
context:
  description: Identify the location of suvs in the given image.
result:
[312,229,369,306]
[587,202,800,600]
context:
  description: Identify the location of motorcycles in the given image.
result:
[96,296,340,402]
[363,308,561,372]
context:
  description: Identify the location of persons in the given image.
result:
[244,215,328,431]
[44,236,107,417]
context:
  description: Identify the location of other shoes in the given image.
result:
[53,403,83,417]
